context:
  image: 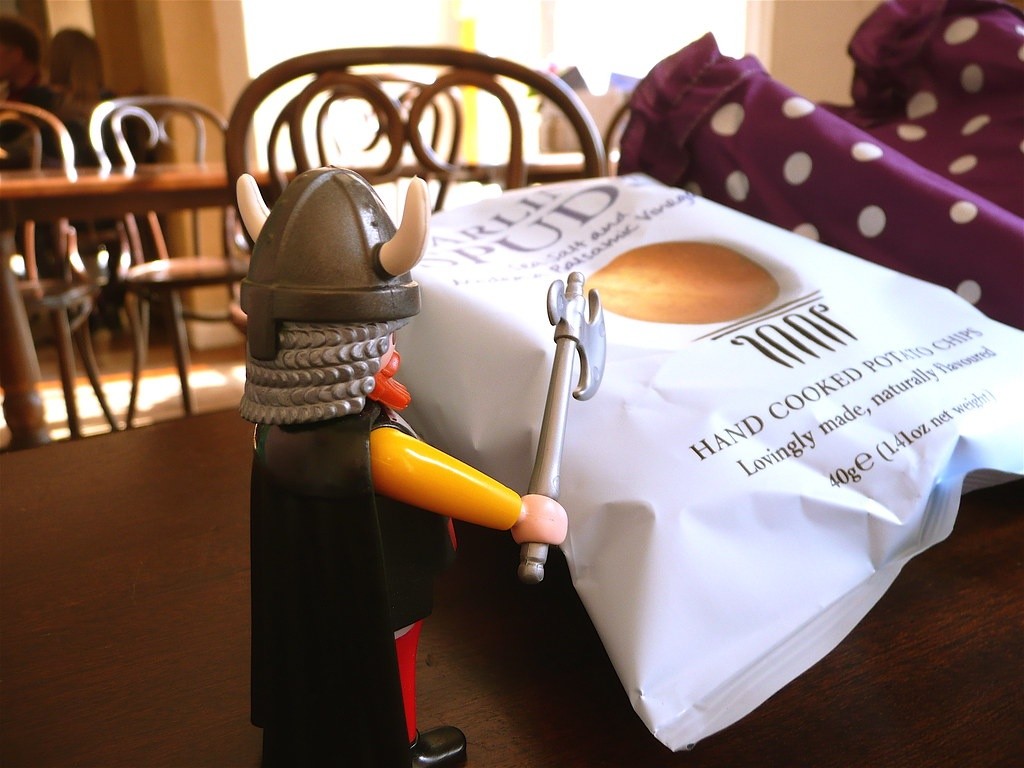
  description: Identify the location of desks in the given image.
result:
[0,150,627,453]
[0,409,1024,768]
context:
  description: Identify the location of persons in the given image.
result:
[0,18,44,99]
[24,30,130,171]
[236,165,569,768]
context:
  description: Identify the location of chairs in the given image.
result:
[0,47,638,455]
